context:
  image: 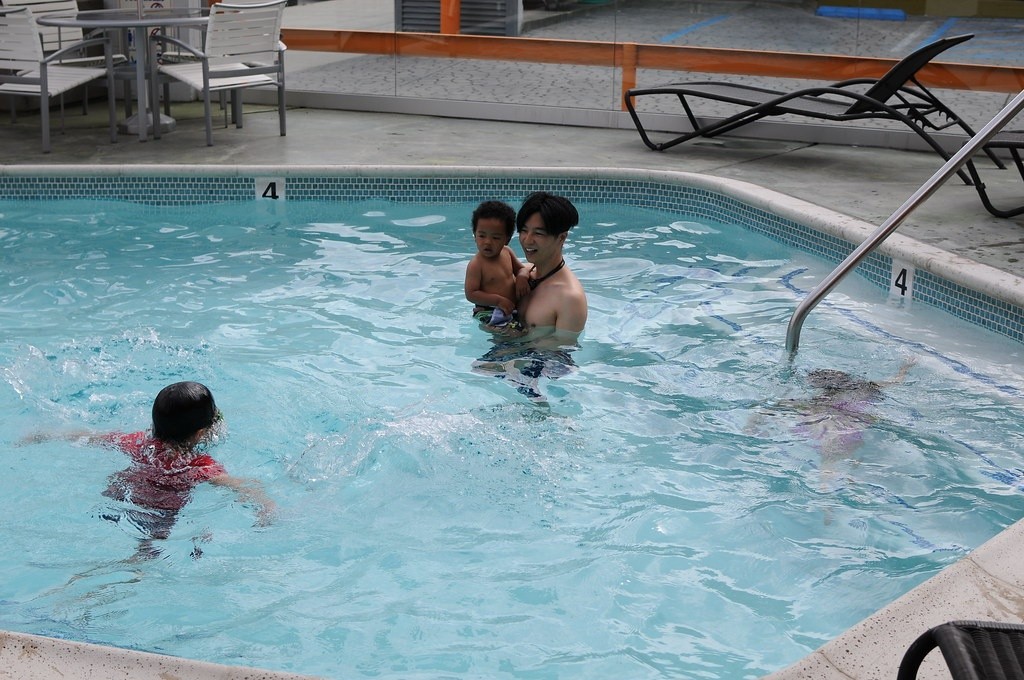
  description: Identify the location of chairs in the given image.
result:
[1,0,133,122]
[148,0,290,146]
[163,0,281,123]
[623,32,1007,186]
[961,139,1023,219]
[0,4,121,154]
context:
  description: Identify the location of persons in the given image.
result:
[465,202,531,328]
[517,192,587,332]
[151,381,217,443]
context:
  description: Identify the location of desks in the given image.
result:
[37,6,217,135]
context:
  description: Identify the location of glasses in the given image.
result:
[527,258,565,290]
